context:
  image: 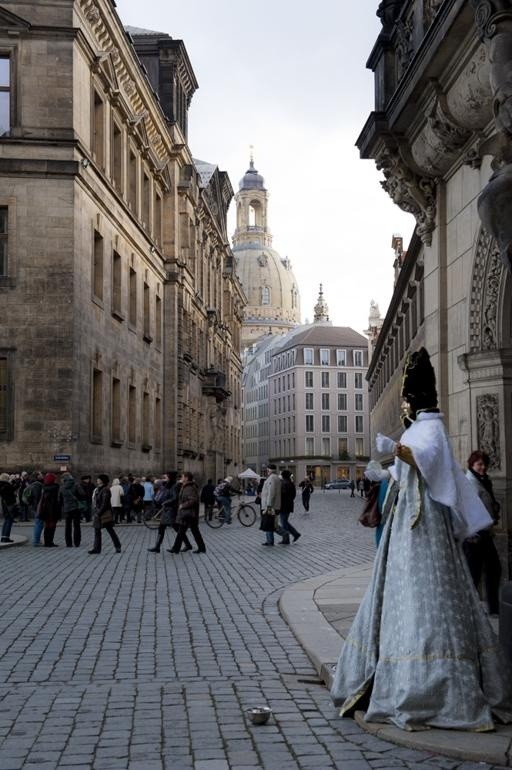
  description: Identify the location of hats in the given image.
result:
[224,476,233,483]
[63,473,73,481]
[268,465,276,468]
[45,474,55,480]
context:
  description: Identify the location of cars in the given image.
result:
[324,478,351,489]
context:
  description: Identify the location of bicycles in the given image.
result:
[142,506,163,532]
[203,488,257,530]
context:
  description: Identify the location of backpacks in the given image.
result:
[100,514,112,525]
[179,509,194,520]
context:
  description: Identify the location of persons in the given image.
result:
[279,470,300,544]
[349,478,372,497]
[1,468,261,554]
[460,450,502,617]
[260,464,290,547]
[330,346,509,731]
[298,476,313,512]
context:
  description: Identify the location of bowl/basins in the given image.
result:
[244,706,273,724]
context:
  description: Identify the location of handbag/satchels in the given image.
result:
[359,499,381,528]
[260,513,279,531]
[214,483,226,496]
[78,501,88,511]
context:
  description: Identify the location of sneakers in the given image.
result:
[88,549,100,554]
[167,549,179,554]
[1,537,14,542]
[193,550,206,554]
[182,546,192,551]
[148,548,160,553]
[294,534,300,542]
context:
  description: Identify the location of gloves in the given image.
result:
[365,469,388,482]
[375,433,395,455]
